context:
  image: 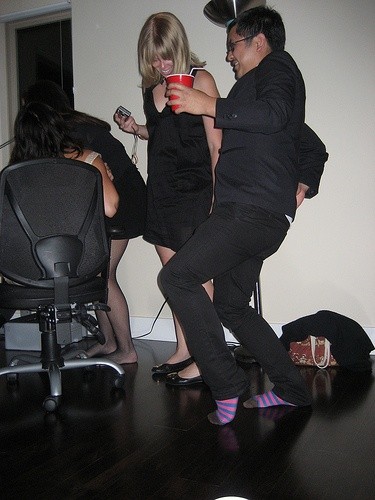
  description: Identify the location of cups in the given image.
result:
[165,72,195,112]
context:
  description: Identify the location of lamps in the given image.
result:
[203,0,267,363]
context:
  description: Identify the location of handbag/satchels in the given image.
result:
[288,334,340,368]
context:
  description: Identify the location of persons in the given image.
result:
[6,100,120,219]
[112,10,222,387]
[19,79,148,367]
[156,5,327,427]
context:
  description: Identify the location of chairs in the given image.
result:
[0,157,126,412]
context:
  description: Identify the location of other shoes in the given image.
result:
[165,371,203,385]
[153,355,193,373]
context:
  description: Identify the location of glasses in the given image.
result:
[227,34,258,53]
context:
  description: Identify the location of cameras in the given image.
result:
[115,106,132,122]
